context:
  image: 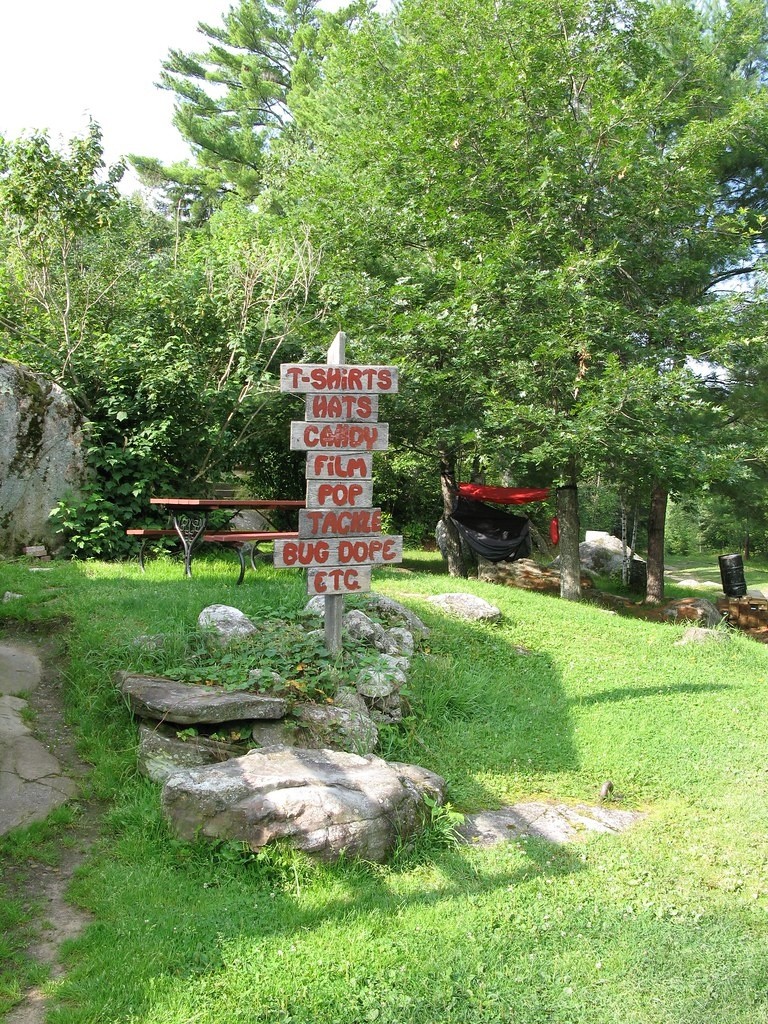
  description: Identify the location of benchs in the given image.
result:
[205,531,300,587]
[124,528,283,582]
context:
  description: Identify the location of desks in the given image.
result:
[149,498,306,580]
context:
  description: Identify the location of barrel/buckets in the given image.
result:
[718,553,746,596]
